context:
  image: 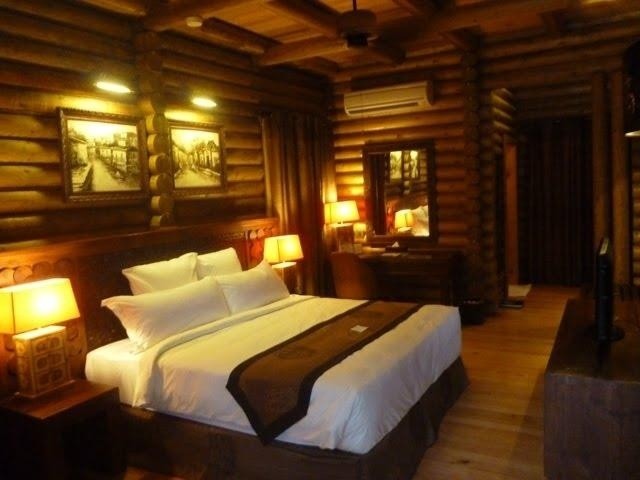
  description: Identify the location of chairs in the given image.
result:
[330,252,380,300]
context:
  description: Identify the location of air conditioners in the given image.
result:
[344,81,434,120]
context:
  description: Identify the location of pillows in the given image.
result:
[216,260,289,312]
[101,277,230,355]
[122,251,197,295]
[197,247,243,280]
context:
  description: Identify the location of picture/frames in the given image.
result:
[168,119,228,200]
[56,107,149,209]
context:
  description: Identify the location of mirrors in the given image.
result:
[363,139,437,246]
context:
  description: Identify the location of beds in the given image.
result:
[0,216,470,480]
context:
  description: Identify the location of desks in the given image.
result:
[360,243,458,307]
[543,296,640,478]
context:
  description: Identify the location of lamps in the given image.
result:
[325,201,361,251]
[0,277,82,401]
[263,234,304,282]
[394,208,413,231]
[334,0,381,51]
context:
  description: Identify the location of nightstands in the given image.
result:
[0,381,120,480]
